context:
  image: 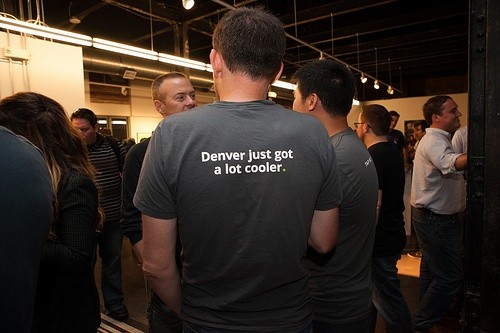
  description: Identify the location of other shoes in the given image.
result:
[407,251,421,260]
[110,303,128,322]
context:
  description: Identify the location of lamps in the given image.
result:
[360,72,368,84]
[182,0,195,10]
[374,80,380,90]
[387,86,394,95]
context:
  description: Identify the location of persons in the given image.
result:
[133,8,344,333]
[0,59,472,333]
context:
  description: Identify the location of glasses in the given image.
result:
[354,123,371,131]
[416,129,421,132]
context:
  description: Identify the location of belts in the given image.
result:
[418,206,442,217]
